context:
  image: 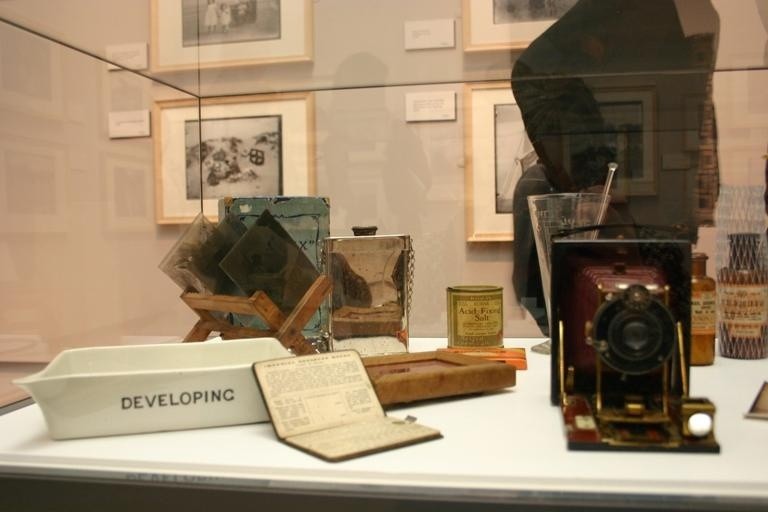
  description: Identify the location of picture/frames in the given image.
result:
[592,84,658,200]
[461,0,579,54]
[151,89,317,225]
[462,80,539,244]
[146,0,314,75]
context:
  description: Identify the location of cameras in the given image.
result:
[551,239,721,454]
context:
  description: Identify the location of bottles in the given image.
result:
[688,250,716,367]
[714,231,768,361]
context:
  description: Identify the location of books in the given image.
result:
[251,349,444,463]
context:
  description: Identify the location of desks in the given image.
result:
[0,337,768,512]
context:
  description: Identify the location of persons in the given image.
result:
[202,0,246,30]
[193,132,245,183]
